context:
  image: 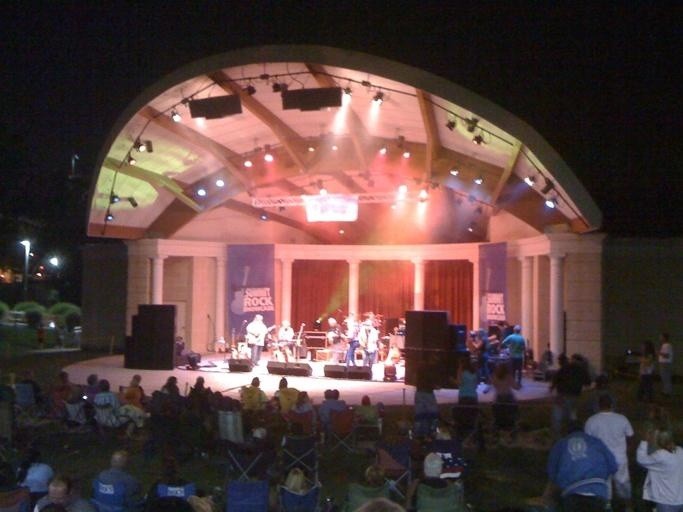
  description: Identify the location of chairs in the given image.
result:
[0,387,612,511]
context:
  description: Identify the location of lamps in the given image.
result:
[525,176,536,186]
[112,195,120,203]
[196,139,483,235]
[374,92,384,104]
[171,111,181,122]
[446,120,456,130]
[107,214,113,221]
[247,85,256,96]
[271,82,281,93]
[343,87,352,100]
[546,197,555,208]
[473,134,483,144]
[128,156,136,165]
[136,142,146,151]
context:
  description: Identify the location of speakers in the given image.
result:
[324,365,346,378]
[229,359,253,372]
[267,361,287,374]
[346,366,373,379]
[448,325,470,389]
[405,309,448,389]
[286,363,312,376]
[124,315,138,370]
[138,303,174,370]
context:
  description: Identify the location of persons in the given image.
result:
[0,312,683,512]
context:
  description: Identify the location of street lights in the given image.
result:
[21,239,31,301]
